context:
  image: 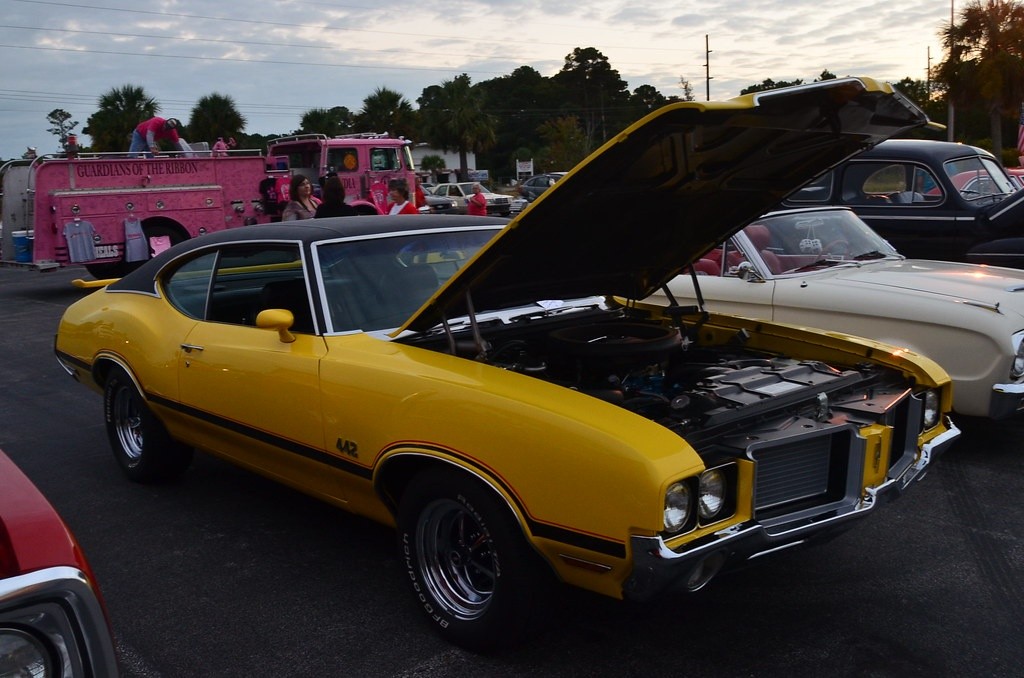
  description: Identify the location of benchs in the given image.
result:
[794,186,858,201]
[179,279,363,325]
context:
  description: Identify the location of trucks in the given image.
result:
[0,133,419,280]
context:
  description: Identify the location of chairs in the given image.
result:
[257,279,315,333]
[887,190,924,204]
[379,263,439,315]
[684,259,720,276]
[857,194,892,205]
[727,225,782,275]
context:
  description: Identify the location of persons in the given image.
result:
[916,161,958,194]
[415,177,426,208]
[281,174,320,221]
[467,183,486,215]
[128,117,185,158]
[313,173,357,217]
[384,178,420,215]
[212,137,240,157]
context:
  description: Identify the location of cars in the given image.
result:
[53,75,966,654]
[517,171,568,203]
[0,451,123,678]
[785,138,1022,272]
[453,182,514,216]
[631,201,1024,426]
[419,184,462,214]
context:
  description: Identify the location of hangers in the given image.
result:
[374,178,380,184]
[283,172,289,178]
[73,212,81,223]
[123,210,142,224]
[268,173,275,178]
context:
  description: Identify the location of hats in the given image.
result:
[165,118,178,130]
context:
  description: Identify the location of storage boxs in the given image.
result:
[11,230,34,262]
